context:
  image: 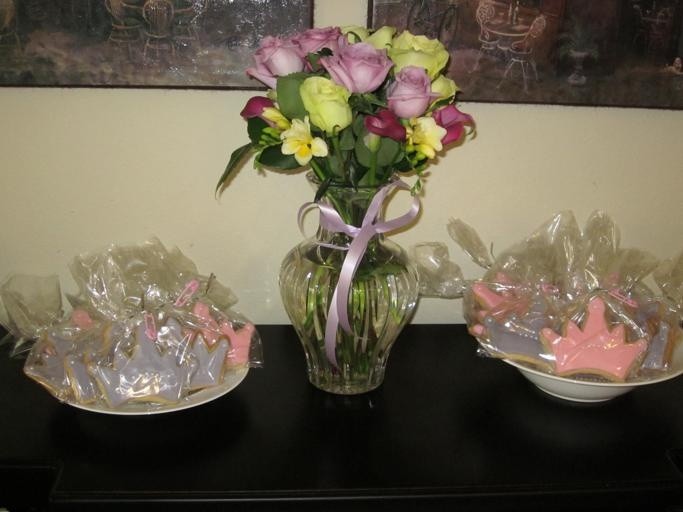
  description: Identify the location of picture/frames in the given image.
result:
[364,1,681,108]
[2,1,319,95]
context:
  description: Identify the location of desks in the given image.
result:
[0,326,683,512]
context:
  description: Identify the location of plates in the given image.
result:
[470,331,682,403]
[23,324,251,416]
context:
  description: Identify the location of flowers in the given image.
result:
[215,24,476,393]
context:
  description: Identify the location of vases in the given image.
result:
[278,171,420,395]
